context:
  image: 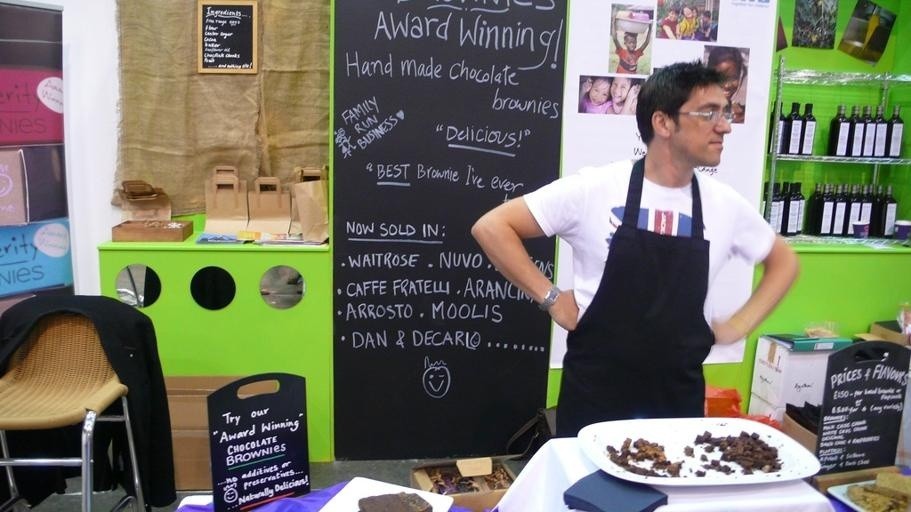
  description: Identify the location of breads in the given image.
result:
[359,494,432,512]
[846,474,911,512]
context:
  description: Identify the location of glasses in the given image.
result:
[678,110,735,125]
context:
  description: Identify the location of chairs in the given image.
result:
[0,298,149,512]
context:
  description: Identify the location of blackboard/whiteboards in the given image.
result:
[815,341,911,476]
[196,0,259,74]
[330,0,570,460]
[206,373,311,511]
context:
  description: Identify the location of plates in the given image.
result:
[313,475,454,512]
[578,416,822,487]
[827,479,910,512]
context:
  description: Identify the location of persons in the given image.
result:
[605,77,641,114]
[611,15,652,75]
[659,5,718,42]
[470,63,799,438]
[578,76,638,115]
[707,46,745,123]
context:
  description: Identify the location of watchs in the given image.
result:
[538,285,562,312]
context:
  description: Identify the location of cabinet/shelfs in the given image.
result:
[770,57,904,237]
[97,218,333,463]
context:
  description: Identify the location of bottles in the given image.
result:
[785,102,803,154]
[801,103,817,155]
[887,106,904,159]
[874,106,889,159]
[861,183,875,230]
[762,181,806,237]
[849,106,864,157]
[875,185,897,238]
[769,101,786,154]
[810,183,849,237]
[844,184,861,236]
[828,106,850,156]
[861,106,876,157]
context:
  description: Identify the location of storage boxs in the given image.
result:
[409,453,525,508]
[784,408,837,448]
[812,468,907,492]
[0,219,78,296]
[165,377,278,493]
[749,395,789,418]
[872,321,911,344]
[1,71,66,145]
[2,149,67,224]
[748,332,860,403]
[3,4,65,68]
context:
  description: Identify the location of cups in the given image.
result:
[895,220,911,239]
[852,222,870,238]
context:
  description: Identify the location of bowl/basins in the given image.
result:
[616,17,648,35]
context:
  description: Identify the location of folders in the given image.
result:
[761,334,853,352]
